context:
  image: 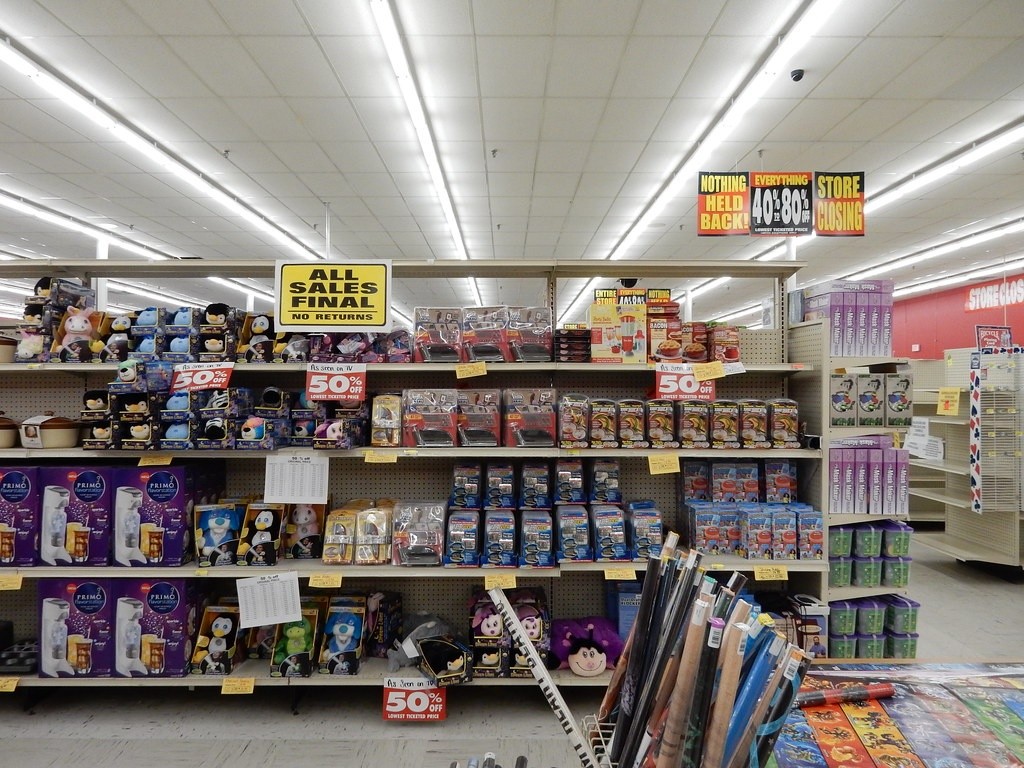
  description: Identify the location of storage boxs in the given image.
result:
[586,303,739,364]
[50,306,553,363]
[677,459,823,561]
[829,523,920,659]
[81,388,800,448]
[0,458,326,567]
[799,279,895,357]
[39,577,551,687]
[769,611,825,656]
[607,575,643,643]
[830,373,912,427]
[555,329,591,363]
[829,434,910,514]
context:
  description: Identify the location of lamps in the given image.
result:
[558,0,1023,329]
[0,0,482,326]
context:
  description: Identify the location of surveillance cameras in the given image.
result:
[791,70,804,81]
[620,278,637,288]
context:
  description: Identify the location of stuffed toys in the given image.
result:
[441,605,624,677]
[16,277,359,442]
[191,612,361,663]
[388,612,451,673]
[197,505,319,558]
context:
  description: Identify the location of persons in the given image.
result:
[215,543,233,565]
[810,637,826,658]
[66,343,81,359]
[286,342,306,363]
[286,656,302,676]
[251,545,269,563]
[204,653,225,675]
[250,342,265,361]
[333,654,350,674]
[105,343,120,362]
[297,538,312,557]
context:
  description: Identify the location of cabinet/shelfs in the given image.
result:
[0,260,910,710]
[904,344,1024,585]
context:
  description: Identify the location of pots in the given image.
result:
[0,410,17,449]
[16,411,79,448]
[0,331,18,363]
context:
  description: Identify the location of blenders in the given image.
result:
[620,316,635,355]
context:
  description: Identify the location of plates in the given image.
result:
[682,355,707,361]
[723,356,739,361]
[653,351,681,359]
